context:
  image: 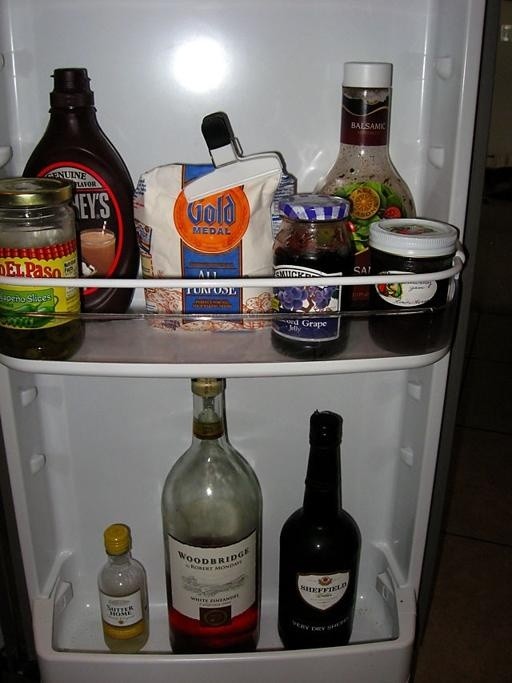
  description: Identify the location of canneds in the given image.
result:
[0,180,87,361]
[272,194,358,358]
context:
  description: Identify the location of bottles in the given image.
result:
[273,408,361,649]
[95,520,150,654]
[158,375,265,652]
[26,65,139,321]
[308,59,420,320]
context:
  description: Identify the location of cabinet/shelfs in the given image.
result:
[4,1,489,682]
